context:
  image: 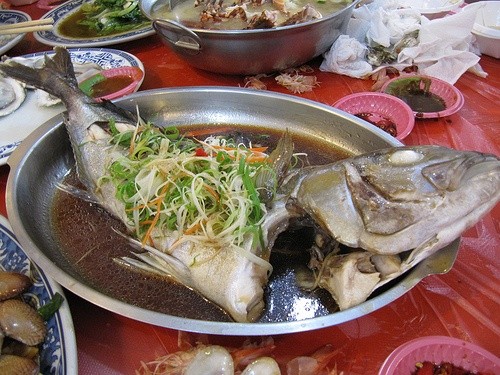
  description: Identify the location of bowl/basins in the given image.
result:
[462,0,500,59]
[373,1,464,20]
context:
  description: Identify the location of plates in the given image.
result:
[378,336,500,375]
[330,92,415,141]
[6,86,461,336]
[0,48,145,166]
[0,214,78,375]
[33,0,156,47]
[37,0,58,10]
[381,74,464,118]
[0,9,32,54]
[79,66,144,103]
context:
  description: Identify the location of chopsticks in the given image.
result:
[1,18,54,35]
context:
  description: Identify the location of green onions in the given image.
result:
[95,117,299,250]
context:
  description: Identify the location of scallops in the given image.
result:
[0,272,45,375]
[183,345,281,375]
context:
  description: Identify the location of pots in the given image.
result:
[138,0,365,76]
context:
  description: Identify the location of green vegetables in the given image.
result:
[75,0,155,37]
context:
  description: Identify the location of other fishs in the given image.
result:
[0,47,500,323]
